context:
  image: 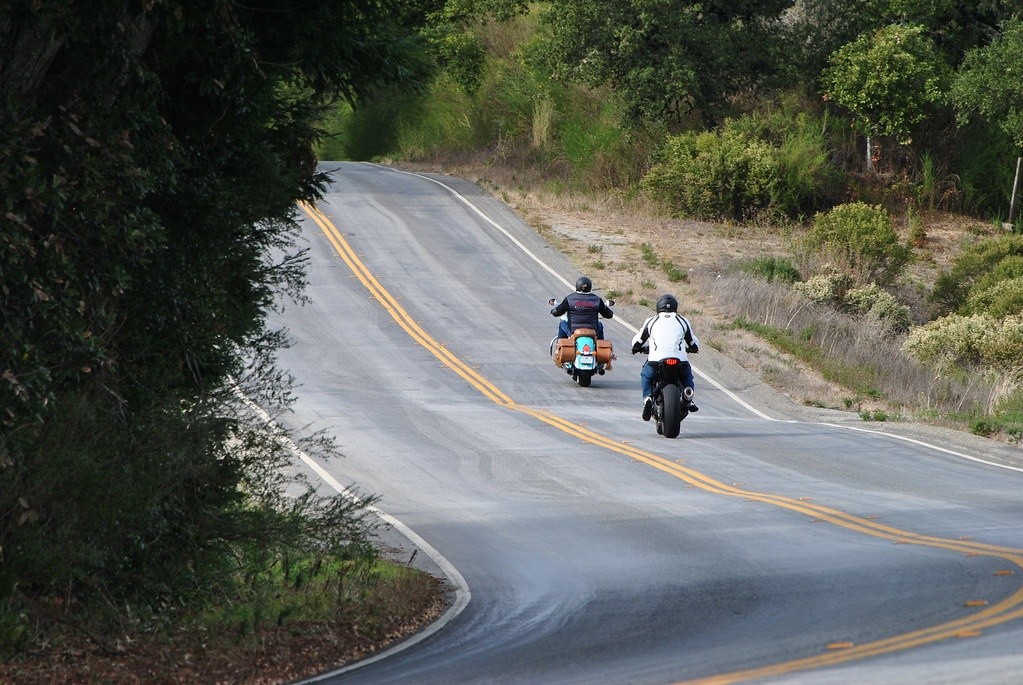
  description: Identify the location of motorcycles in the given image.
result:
[635,346,699,438]
[548,298,615,387]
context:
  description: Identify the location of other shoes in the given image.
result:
[642,397,652,421]
[690,403,698,412]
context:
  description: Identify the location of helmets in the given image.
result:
[656,294,677,312]
[576,277,592,292]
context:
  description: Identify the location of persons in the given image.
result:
[632,294,699,421]
[551,277,613,369]
